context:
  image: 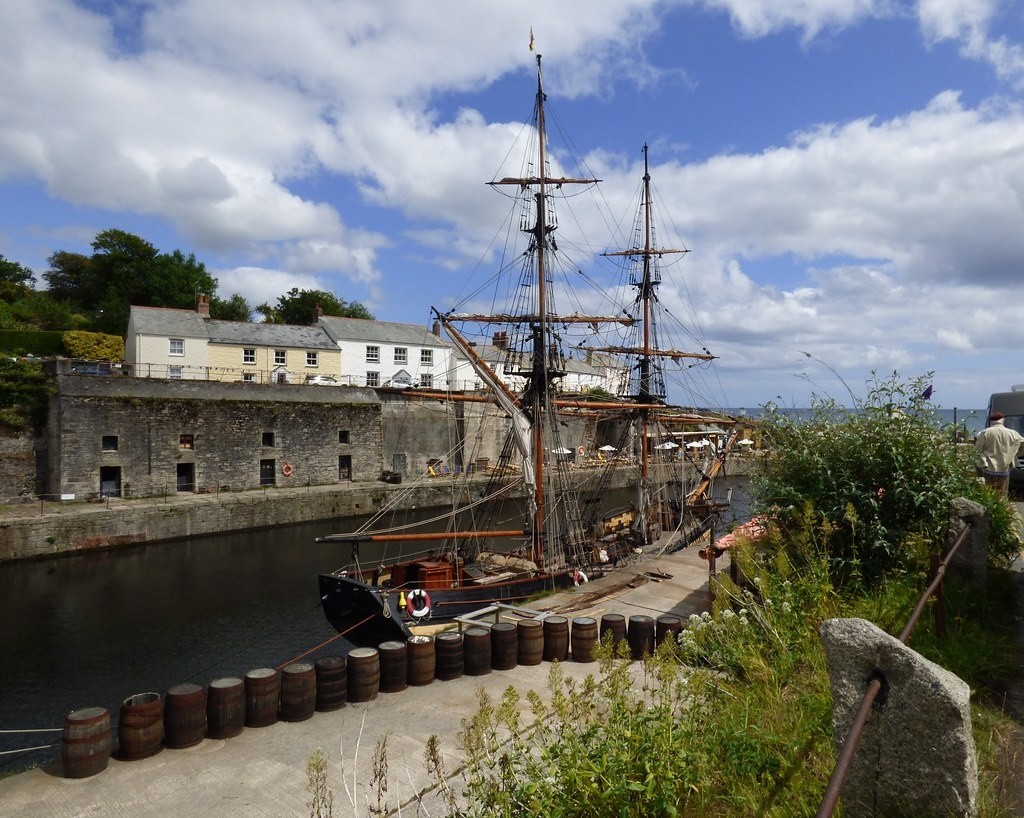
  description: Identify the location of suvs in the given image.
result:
[303,375,349,386]
[382,378,420,389]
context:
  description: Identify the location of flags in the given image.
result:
[529,32,534,51]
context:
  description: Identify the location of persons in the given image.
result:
[971,412,1024,499]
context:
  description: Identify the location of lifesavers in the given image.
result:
[282,464,293,476]
[407,588,431,618]
[573,570,589,586]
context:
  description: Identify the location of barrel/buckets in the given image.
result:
[118,692,163,760]
[206,615,682,741]
[62,707,111,778]
[163,683,206,749]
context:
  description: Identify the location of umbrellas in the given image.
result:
[654,440,709,449]
[738,439,754,444]
[599,445,617,450]
[552,447,571,453]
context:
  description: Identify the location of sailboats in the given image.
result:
[312,54,747,655]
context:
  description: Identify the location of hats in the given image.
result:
[990,412,1004,421]
